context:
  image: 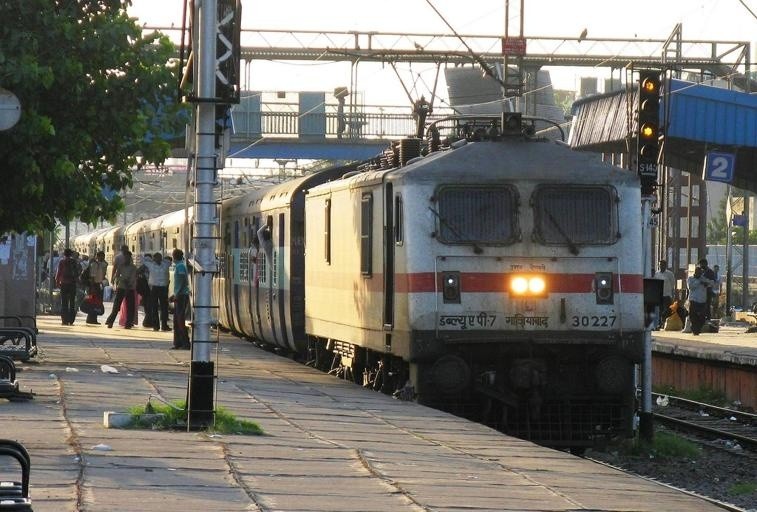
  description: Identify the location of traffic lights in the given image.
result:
[639,69,660,160]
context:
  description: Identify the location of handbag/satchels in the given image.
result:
[80,293,105,316]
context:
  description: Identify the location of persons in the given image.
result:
[698,258,715,320]
[249,238,260,289]
[685,266,714,336]
[256,222,273,271]
[653,260,676,330]
[40,243,173,332]
[166,248,192,351]
[710,264,723,319]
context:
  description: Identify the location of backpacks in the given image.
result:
[80,264,91,281]
[135,264,149,297]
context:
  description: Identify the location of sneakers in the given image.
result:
[170,343,191,350]
[61,319,173,332]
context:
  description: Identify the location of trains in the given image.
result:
[53,112,644,456]
